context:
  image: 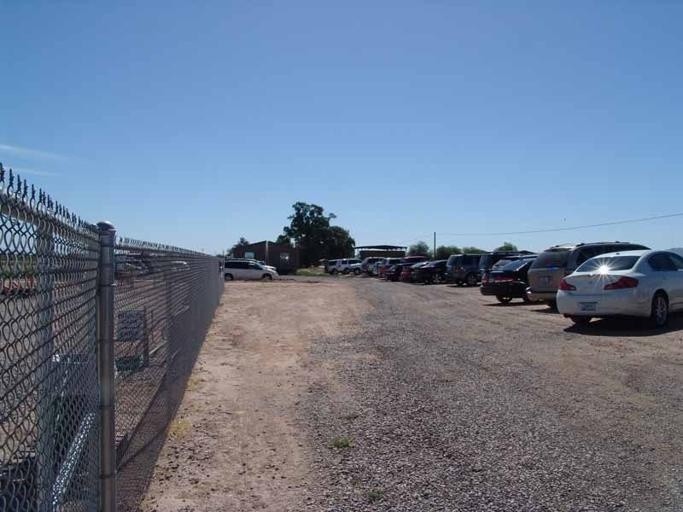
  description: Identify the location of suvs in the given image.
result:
[476,250,534,279]
[446,254,482,287]
[525,240,652,312]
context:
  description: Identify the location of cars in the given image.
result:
[114,251,280,281]
[480,255,540,304]
[324,256,448,285]
[555,249,683,328]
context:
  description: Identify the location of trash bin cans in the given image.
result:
[52,353,94,500]
